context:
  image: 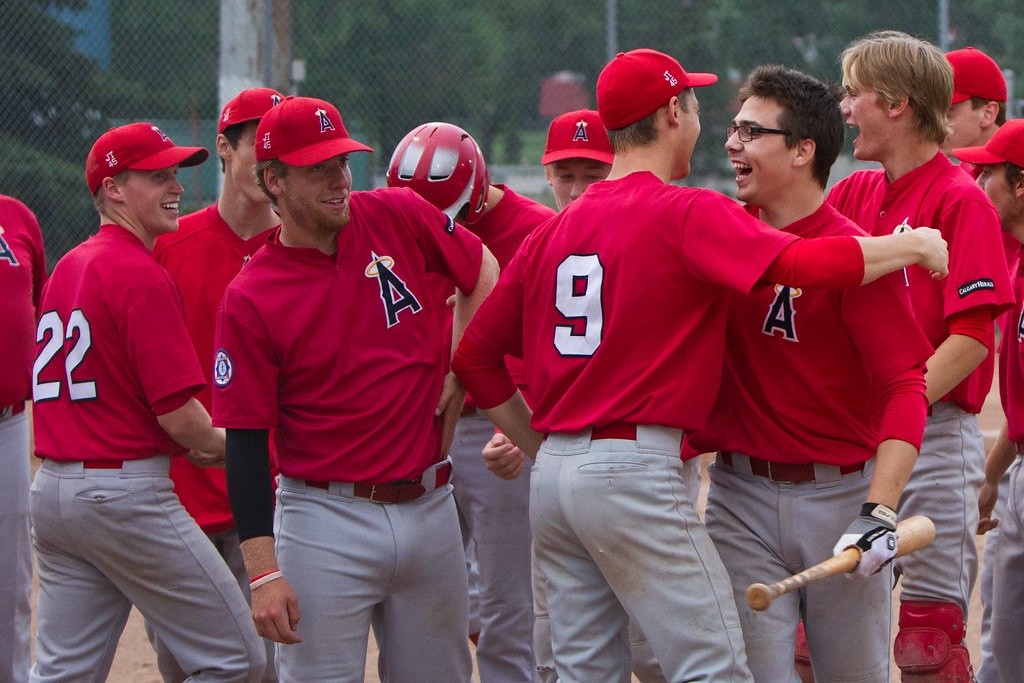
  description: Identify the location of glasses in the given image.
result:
[725,125,797,142]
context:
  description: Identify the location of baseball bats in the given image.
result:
[744,514,937,613]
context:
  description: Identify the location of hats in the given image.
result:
[596,48,718,130]
[541,109,615,164]
[951,118,1024,167]
[85,121,209,197]
[255,96,374,167]
[218,88,286,135]
[944,46,1008,105]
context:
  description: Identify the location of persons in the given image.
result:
[151,89,281,683]
[704,66,936,683]
[826,35,1024,683]
[388,122,556,683]
[543,109,701,683]
[0,193,48,683]
[451,49,948,683]
[28,123,267,683]
[213,97,500,683]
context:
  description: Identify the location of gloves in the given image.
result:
[832,502,897,580]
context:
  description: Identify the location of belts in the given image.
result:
[543,424,636,439]
[11,398,25,417]
[1016,442,1024,455]
[720,452,864,485]
[82,459,124,469]
[927,404,933,417]
[305,463,450,505]
[460,406,478,419]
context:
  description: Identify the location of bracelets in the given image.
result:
[249,570,282,590]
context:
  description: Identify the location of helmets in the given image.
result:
[386,123,489,226]
